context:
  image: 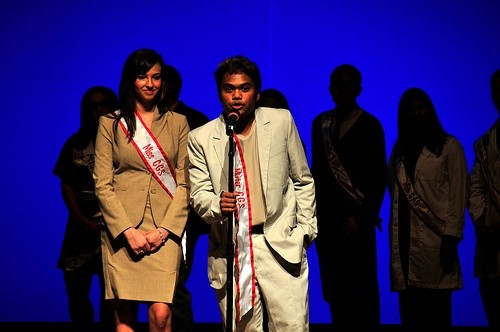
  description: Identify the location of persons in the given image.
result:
[52,48,500,332]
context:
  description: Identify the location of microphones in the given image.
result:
[225,109,240,136]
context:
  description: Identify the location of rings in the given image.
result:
[140,249,144,254]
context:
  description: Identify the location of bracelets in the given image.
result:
[157,228,165,244]
